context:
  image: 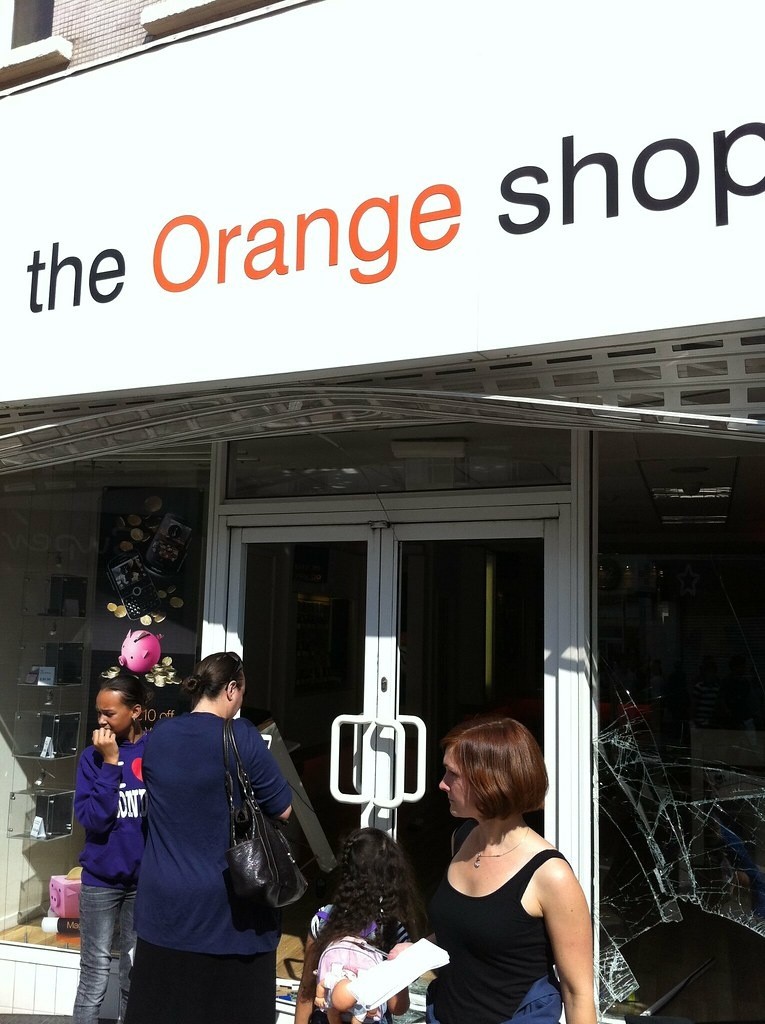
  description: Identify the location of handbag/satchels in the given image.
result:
[223,717,309,908]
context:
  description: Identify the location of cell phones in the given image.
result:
[107,513,193,620]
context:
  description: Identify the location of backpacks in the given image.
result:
[313,910,387,1024]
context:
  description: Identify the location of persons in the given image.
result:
[294,827,427,1024]
[688,655,751,730]
[314,970,377,1024]
[388,716,597,1024]
[72,675,149,1024]
[123,652,292,1024]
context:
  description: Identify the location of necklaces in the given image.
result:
[470,827,529,868]
